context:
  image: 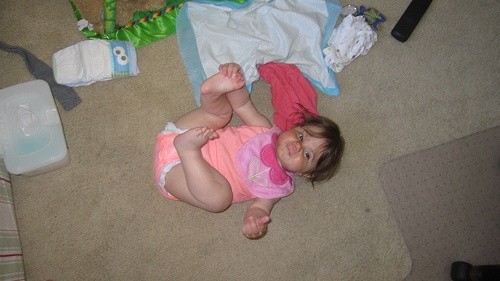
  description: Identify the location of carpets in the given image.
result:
[379,126,500,281]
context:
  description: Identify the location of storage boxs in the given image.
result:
[0,79,70,176]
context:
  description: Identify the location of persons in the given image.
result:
[153,63,344,239]
